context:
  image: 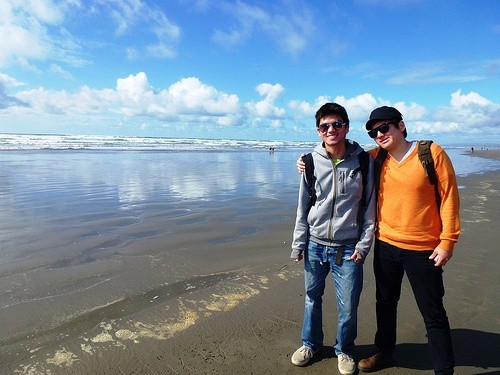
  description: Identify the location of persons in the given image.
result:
[290,102,375,375]
[296,106,461,375]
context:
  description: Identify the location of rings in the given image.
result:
[446,258,449,262]
[355,260,358,262]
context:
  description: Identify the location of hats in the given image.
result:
[365,107,403,131]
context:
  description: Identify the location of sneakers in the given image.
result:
[337,351,356,374]
[292,345,320,365]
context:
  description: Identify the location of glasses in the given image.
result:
[367,122,395,139]
[316,122,346,132]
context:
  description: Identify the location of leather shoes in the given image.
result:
[358,352,395,372]
